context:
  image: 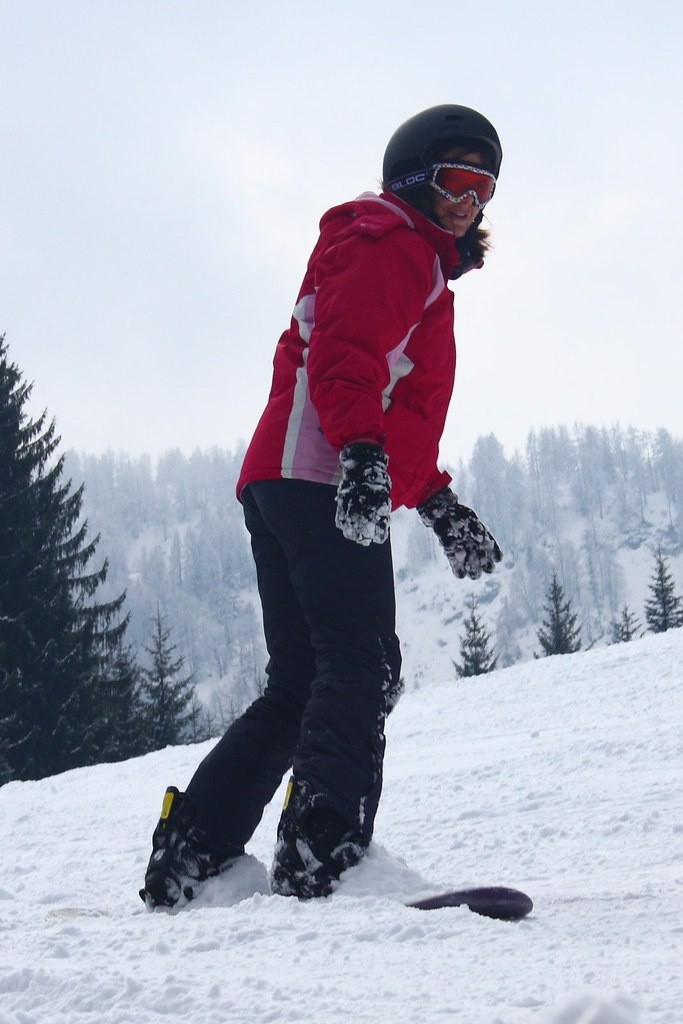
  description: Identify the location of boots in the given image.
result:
[268,778,374,919]
[139,786,244,905]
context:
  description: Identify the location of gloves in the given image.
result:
[415,487,503,580]
[334,441,392,546]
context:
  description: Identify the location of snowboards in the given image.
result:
[135,884,536,931]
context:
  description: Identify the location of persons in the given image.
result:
[139,105,504,910]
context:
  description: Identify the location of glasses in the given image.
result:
[382,158,496,209]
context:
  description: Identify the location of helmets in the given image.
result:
[382,104,502,229]
